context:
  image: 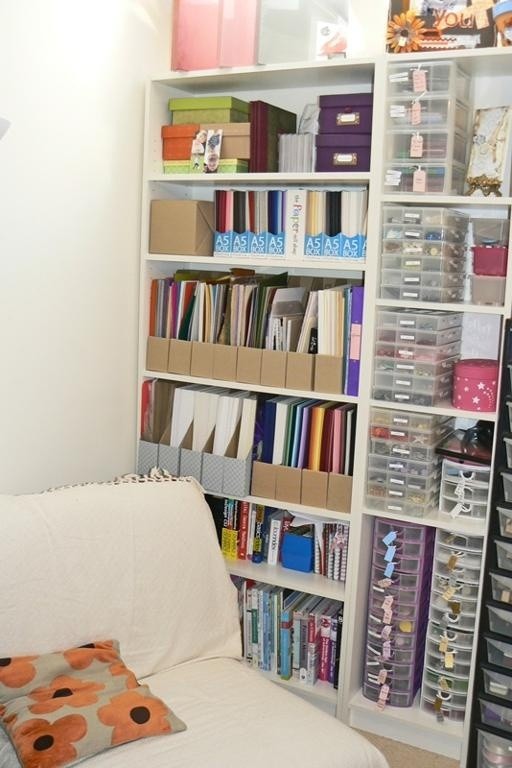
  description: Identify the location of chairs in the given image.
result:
[0,481,396,767]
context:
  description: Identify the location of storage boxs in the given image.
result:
[374,69,478,401]
[359,414,492,734]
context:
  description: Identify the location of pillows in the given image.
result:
[1,486,247,669]
[0,641,191,766]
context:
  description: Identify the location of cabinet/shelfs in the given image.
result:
[131,59,382,721]
[341,51,511,768]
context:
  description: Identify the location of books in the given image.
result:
[231,574,344,689]
[248,100,316,173]
[148,190,363,359]
[142,376,359,581]
